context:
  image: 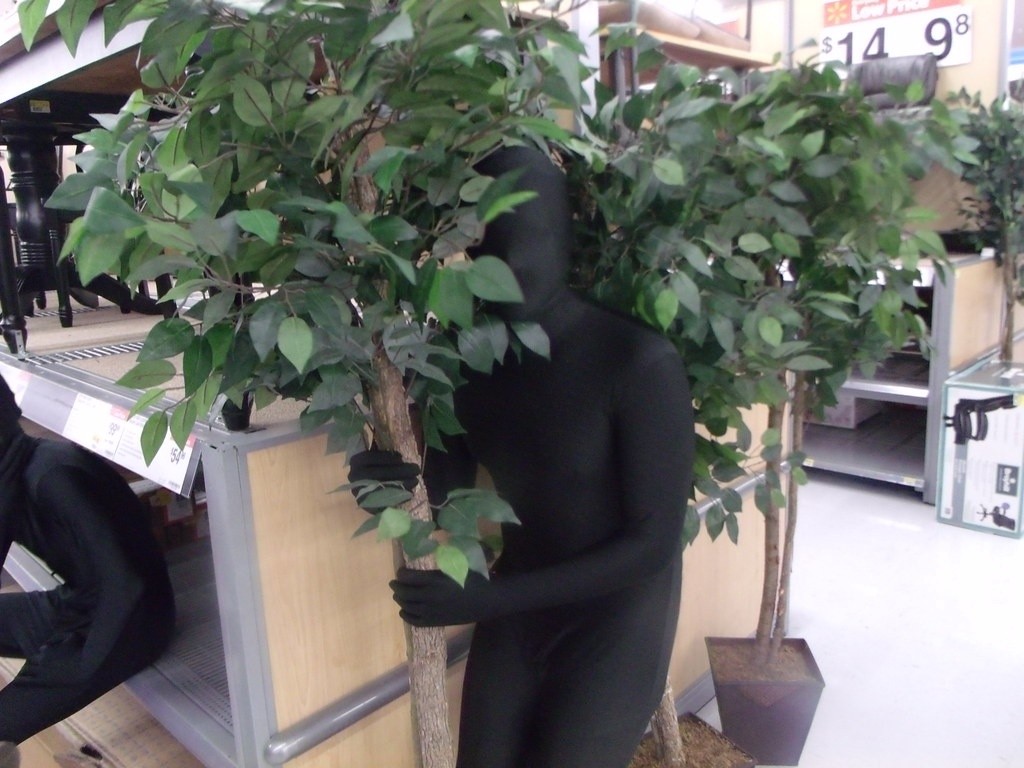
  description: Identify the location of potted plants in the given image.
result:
[920,73,1024,375]
[557,13,976,761]
[10,2,601,768]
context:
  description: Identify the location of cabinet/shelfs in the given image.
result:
[681,0,1021,505]
[3,1,798,768]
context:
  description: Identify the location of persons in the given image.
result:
[0,376,180,751]
[344,148,692,767]
